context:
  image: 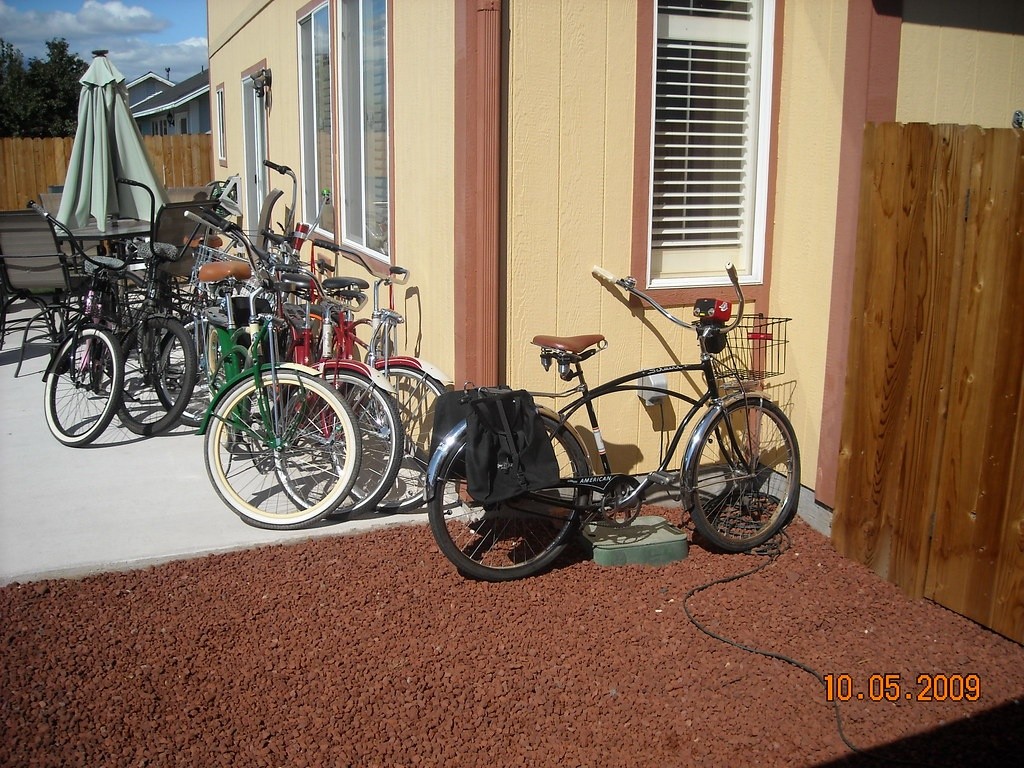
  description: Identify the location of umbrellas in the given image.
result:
[55,56,166,232]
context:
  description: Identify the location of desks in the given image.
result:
[55,219,154,258]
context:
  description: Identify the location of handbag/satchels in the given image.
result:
[429,391,479,479]
[466,388,562,502]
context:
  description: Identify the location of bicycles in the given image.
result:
[424,261,800,581]
[1,160,450,532]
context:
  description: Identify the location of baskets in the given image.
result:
[150,271,217,318]
[191,245,275,312]
[90,279,151,328]
[693,315,792,380]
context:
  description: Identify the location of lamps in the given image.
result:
[250,69,272,98]
[166,110,175,127]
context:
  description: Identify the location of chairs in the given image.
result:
[0,210,88,378]
[119,199,221,282]
[38,193,84,287]
